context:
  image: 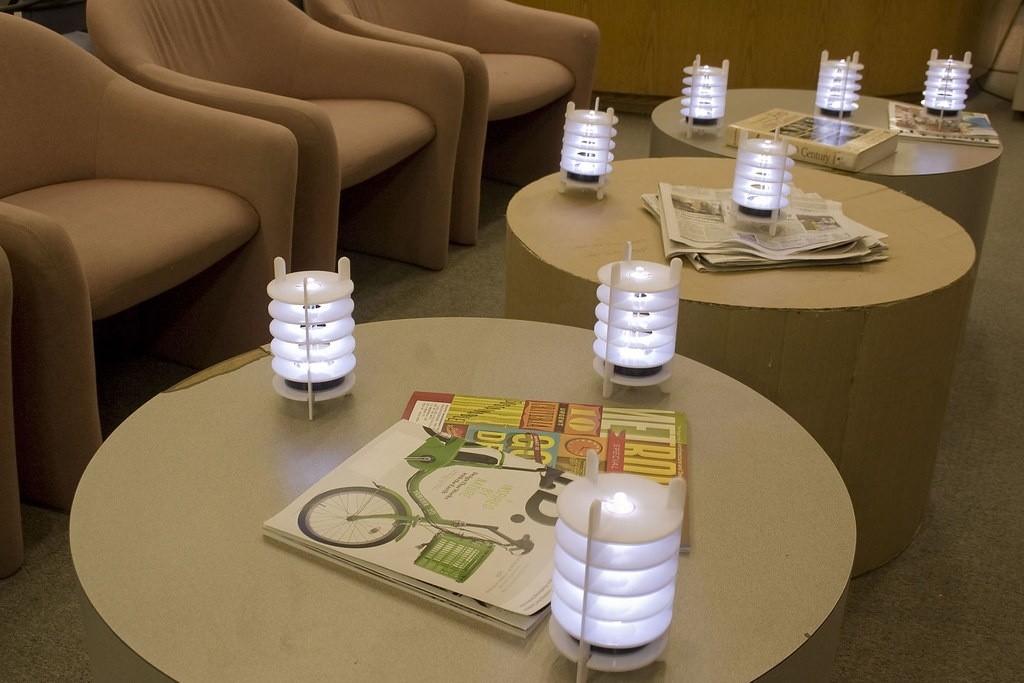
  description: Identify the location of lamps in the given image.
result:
[558,99,618,202]
[270,251,356,418]
[919,47,973,122]
[681,56,730,139]
[591,236,683,411]
[814,48,863,124]
[551,451,687,679]
[728,122,795,239]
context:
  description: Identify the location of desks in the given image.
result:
[648,88,1003,271]
[68,316,858,683]
[501,158,978,581]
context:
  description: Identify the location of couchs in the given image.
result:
[0,12,300,508]
[306,0,600,246]
[86,0,465,274]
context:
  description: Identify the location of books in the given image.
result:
[725,108,900,173]
[263,391,692,642]
[887,103,1000,148]
